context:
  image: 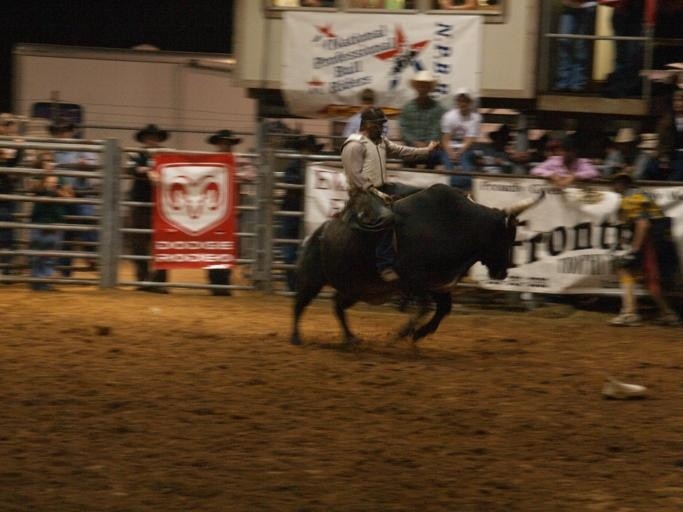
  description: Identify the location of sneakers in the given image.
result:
[380,269,398,281]
[608,311,681,326]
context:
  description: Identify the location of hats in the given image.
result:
[48,115,75,131]
[409,71,436,91]
[137,124,166,142]
[614,127,638,143]
[294,135,324,152]
[209,130,240,145]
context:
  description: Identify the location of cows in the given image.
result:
[287,189,546,346]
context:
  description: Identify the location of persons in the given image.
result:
[600,172,682,326]
[396,63,683,310]
[341,106,441,283]
[203,129,243,296]
[124,123,170,295]
[0,112,97,290]
[548,1,599,93]
[275,88,389,293]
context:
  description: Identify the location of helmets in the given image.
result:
[360,108,387,141]
